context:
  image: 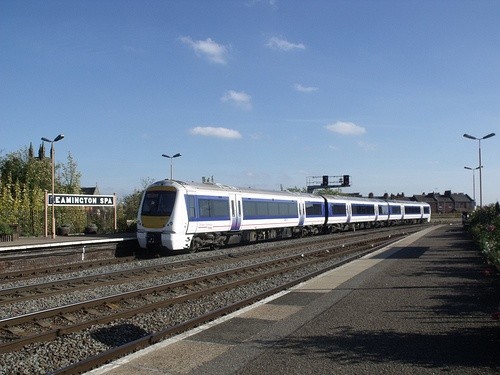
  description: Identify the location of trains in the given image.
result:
[330,189,476,218]
[135,179,432,254]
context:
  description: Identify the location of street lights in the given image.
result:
[463,165,484,201]
[161,153,182,179]
[463,132,496,209]
[40,134,65,239]
